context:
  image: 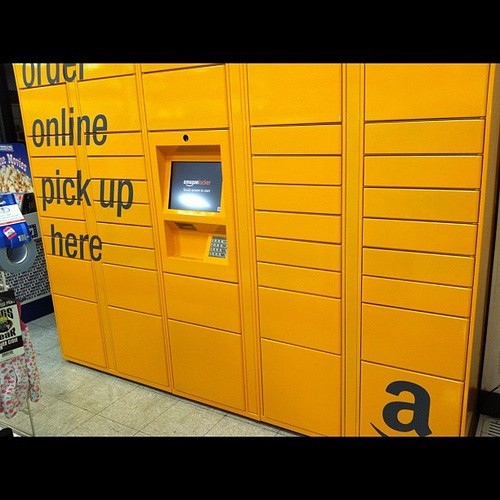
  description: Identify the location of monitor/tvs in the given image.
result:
[168,159,222,213]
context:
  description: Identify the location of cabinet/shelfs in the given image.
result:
[0,318,43,438]
